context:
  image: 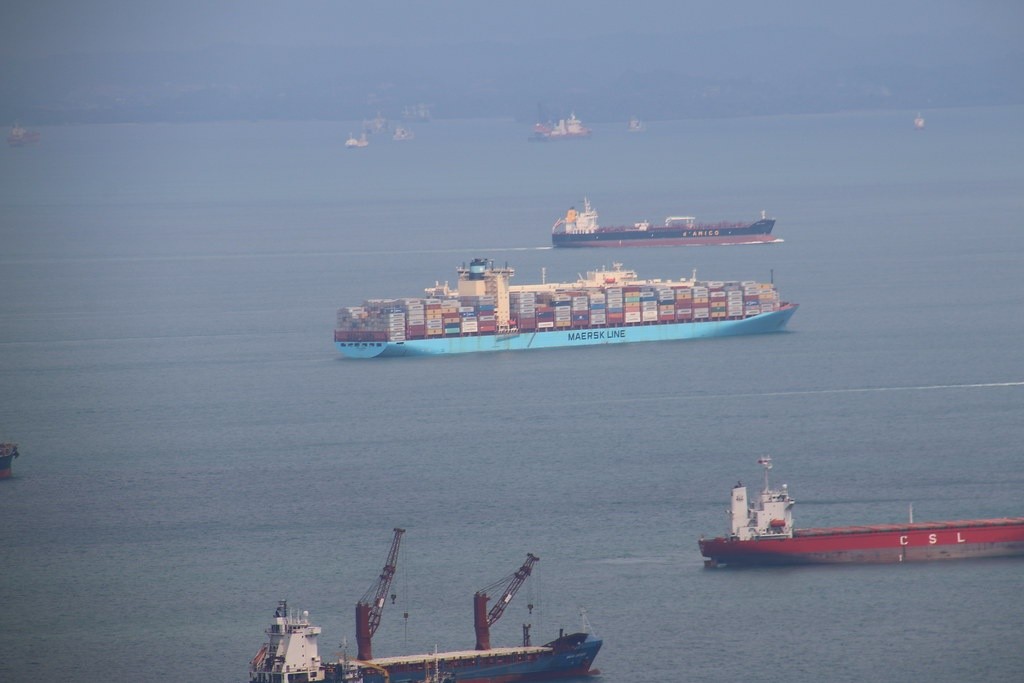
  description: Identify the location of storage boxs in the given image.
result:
[333,278,782,343]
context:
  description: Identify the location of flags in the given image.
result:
[250,644,268,666]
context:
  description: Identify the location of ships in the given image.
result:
[332,253,802,360]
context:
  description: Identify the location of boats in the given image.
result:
[698,454,1024,569]
[0,442,20,480]
[248,528,606,683]
[550,194,785,250]
[528,111,594,141]
